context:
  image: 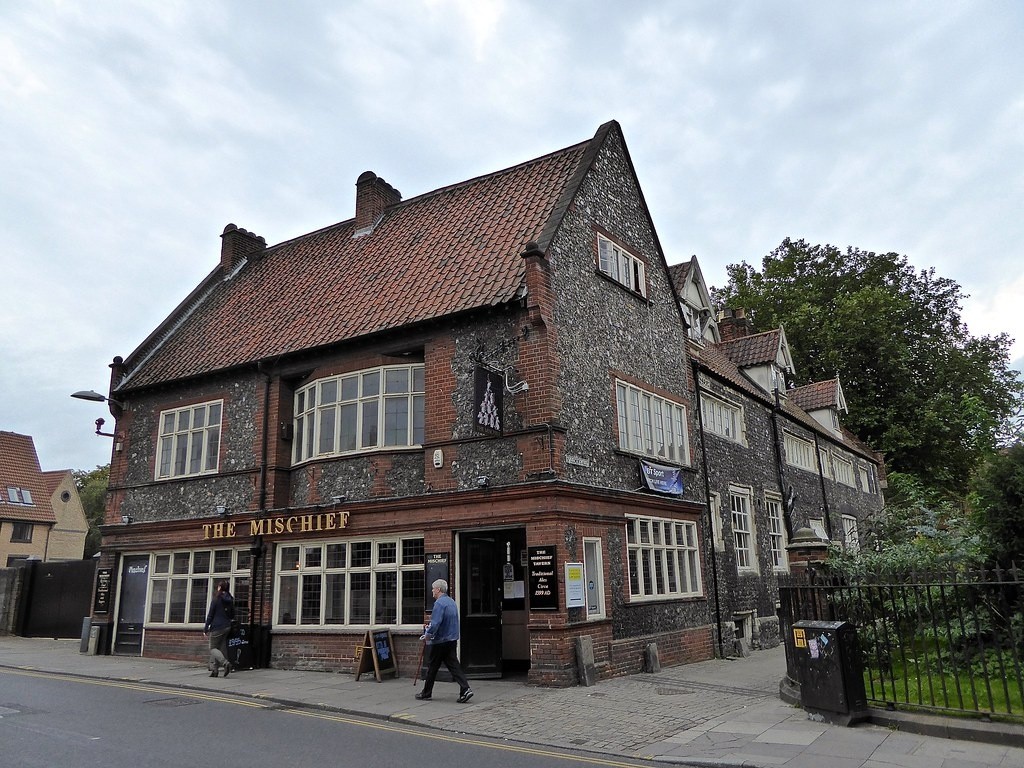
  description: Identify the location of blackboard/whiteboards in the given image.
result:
[354,627,398,675]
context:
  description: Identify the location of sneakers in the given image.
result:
[415,692,432,700]
[457,688,474,703]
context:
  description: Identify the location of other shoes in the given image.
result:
[223,662,234,677]
[209,671,218,677]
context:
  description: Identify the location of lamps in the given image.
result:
[333,493,345,507]
[214,505,230,515]
[71,388,128,412]
[477,475,490,487]
[118,513,137,525]
[93,418,123,438]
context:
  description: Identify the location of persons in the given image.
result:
[415,579,474,703]
[203,581,235,677]
